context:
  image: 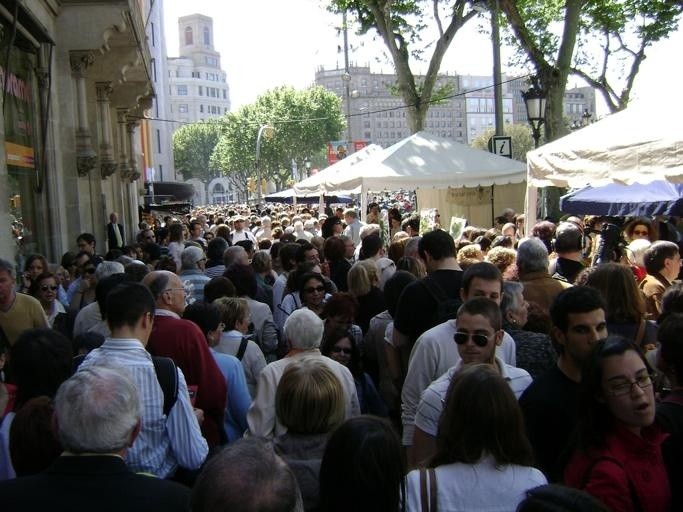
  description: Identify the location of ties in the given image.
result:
[114,225,121,249]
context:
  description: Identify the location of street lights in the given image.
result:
[338,69,360,148]
[253,122,283,201]
[518,73,551,147]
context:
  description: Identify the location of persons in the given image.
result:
[2,202,681,510]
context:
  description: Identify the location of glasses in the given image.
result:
[83,268,96,274]
[304,285,325,293]
[158,287,191,299]
[605,373,657,397]
[454,330,500,347]
[331,345,350,356]
[40,285,58,291]
